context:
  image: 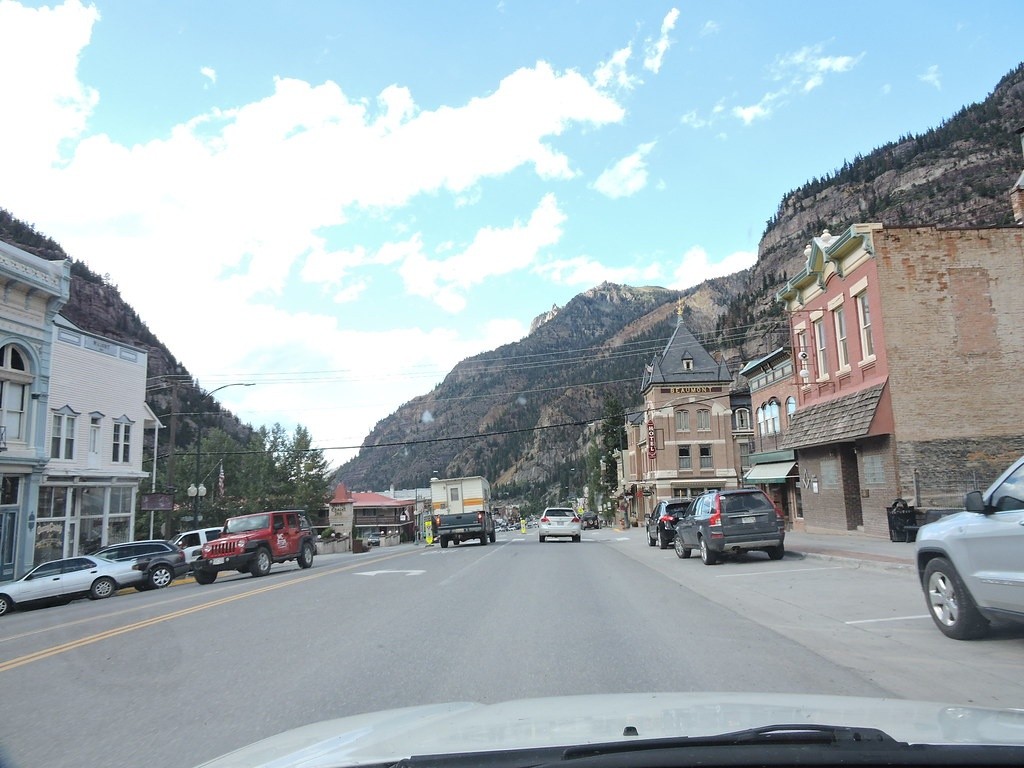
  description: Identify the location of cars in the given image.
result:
[368,532,385,546]
[644,496,696,549]
[0,556,144,616]
[168,526,225,575]
[538,506,581,543]
[495,515,539,532]
[673,486,786,565]
[80,539,190,598]
[582,512,600,530]
[914,454,1024,640]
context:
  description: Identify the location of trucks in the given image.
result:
[430,476,496,548]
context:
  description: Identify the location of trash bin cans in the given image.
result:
[886,498,917,542]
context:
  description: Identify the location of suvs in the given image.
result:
[190,510,318,584]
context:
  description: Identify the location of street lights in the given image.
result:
[192,382,255,532]
